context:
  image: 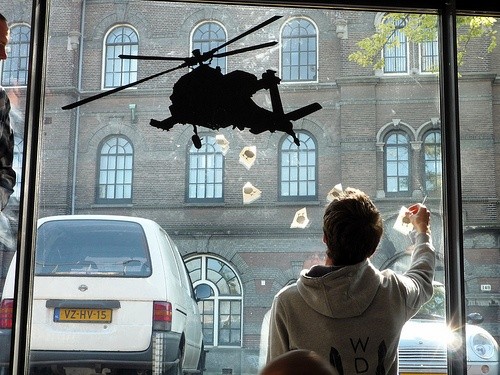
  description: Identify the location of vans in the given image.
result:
[0,214,212,375]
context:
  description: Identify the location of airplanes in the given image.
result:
[60,14,324,146]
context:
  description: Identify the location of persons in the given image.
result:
[0,15,16,213]
[260,349,339,375]
[266,187,436,375]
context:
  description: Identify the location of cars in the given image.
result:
[395,282,500,375]
